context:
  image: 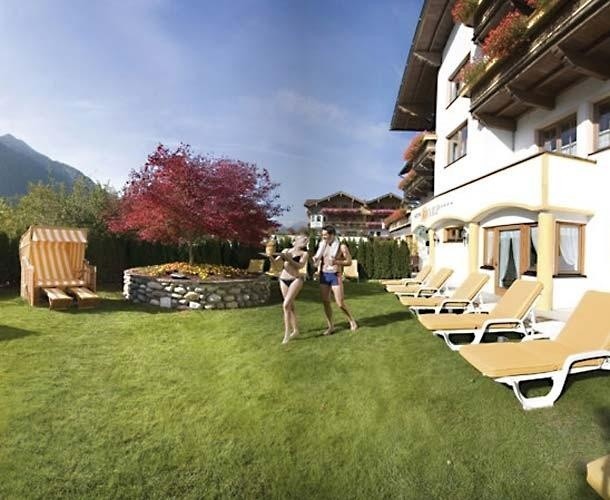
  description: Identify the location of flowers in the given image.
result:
[451,0,579,87]
[385,133,427,229]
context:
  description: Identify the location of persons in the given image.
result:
[257,233,311,345]
[309,224,359,336]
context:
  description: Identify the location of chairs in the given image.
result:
[386,270,455,297]
[398,272,490,319]
[417,278,544,351]
[343,260,360,285]
[459,291,610,410]
[379,267,431,286]
